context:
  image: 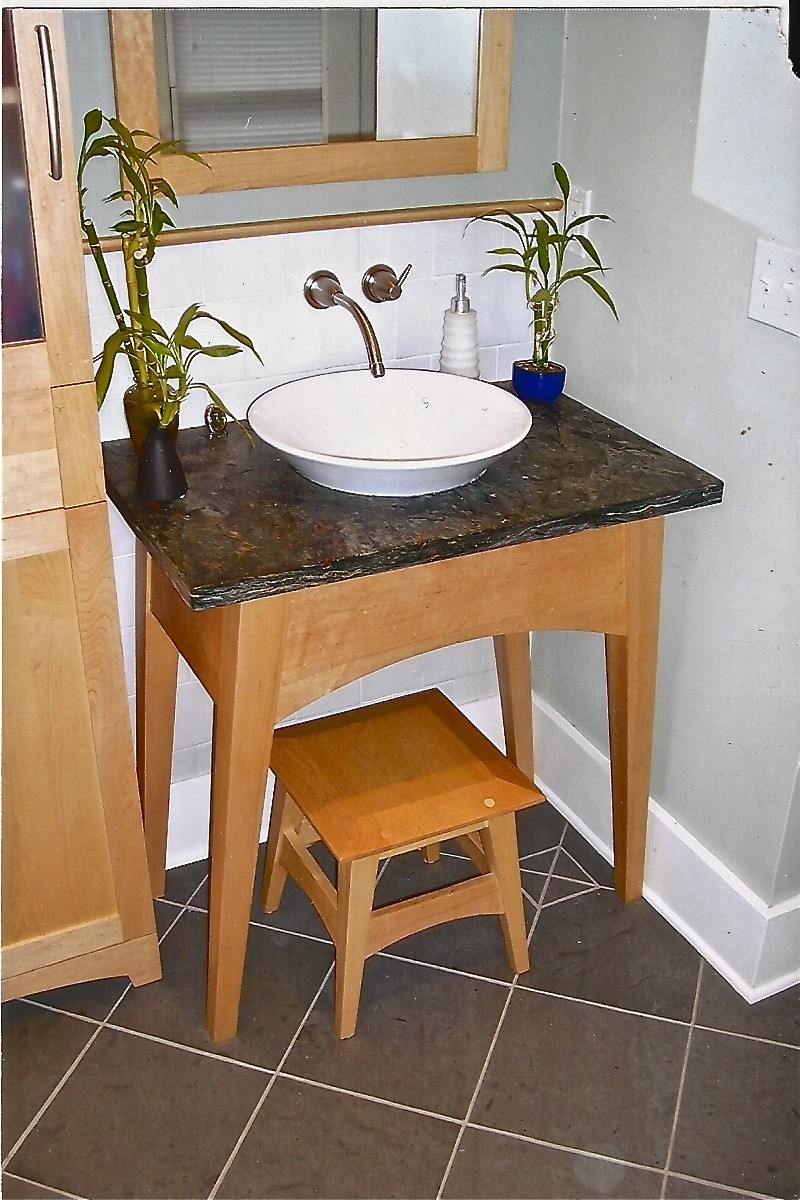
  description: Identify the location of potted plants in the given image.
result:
[463,160,621,409]
[75,109,265,503]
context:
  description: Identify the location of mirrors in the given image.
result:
[108,9,513,202]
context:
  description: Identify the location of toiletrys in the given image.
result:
[438,274,481,380]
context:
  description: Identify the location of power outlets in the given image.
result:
[747,240,800,337]
[564,186,592,261]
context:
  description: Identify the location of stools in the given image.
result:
[261,688,547,1041]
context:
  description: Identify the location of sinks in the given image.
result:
[246,368,532,497]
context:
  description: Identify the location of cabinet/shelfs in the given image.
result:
[2,7,162,1002]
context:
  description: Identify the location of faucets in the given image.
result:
[303,270,385,378]
